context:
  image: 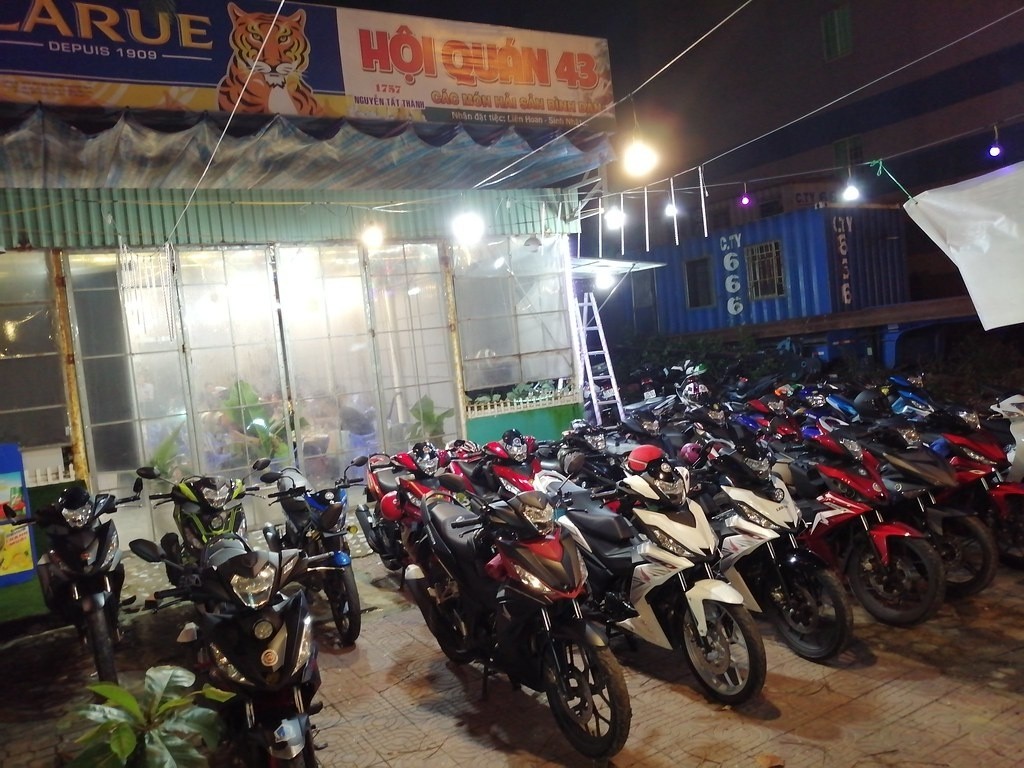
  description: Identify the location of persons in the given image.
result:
[197,397,261,470]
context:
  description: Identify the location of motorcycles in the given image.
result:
[354,341,1024,758]
[129,502,343,768]
[259,454,368,643]
[137,455,272,583]
[1,478,144,687]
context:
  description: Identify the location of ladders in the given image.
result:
[574,292,626,426]
